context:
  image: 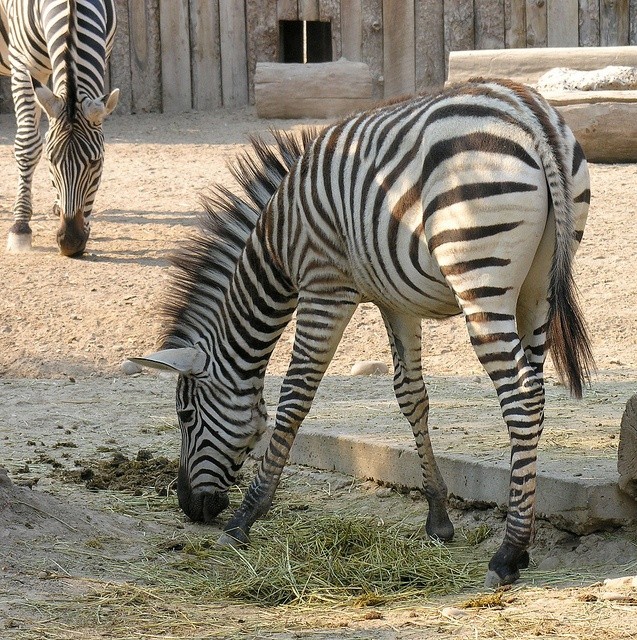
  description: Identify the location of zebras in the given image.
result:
[125,77,598,588]
[0,0,121,257]
